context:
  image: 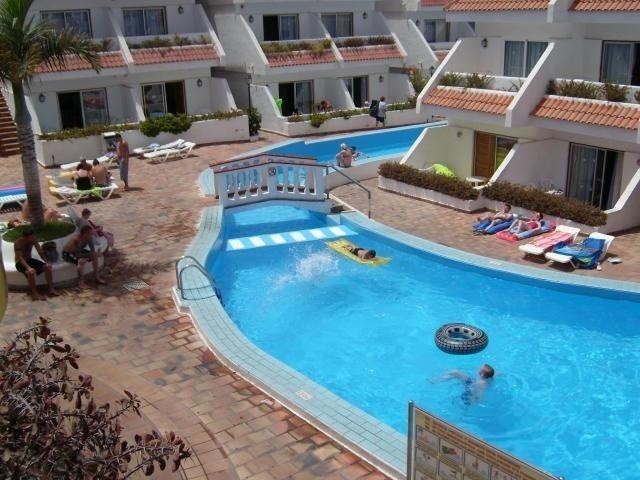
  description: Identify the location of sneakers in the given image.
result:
[607,256,623,263]
[596,265,602,271]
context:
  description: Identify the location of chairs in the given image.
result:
[133,138,197,164]
[518,224,615,268]
[48,151,119,205]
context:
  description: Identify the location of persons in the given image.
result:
[114,133,130,191]
[75,208,117,256]
[503,212,543,237]
[351,146,369,160]
[21,197,67,222]
[62,226,107,290]
[335,143,351,167]
[375,95,387,130]
[476,203,513,234]
[73,164,93,190]
[76,155,92,171]
[89,158,112,187]
[340,241,379,262]
[425,363,502,410]
[13,226,60,300]
